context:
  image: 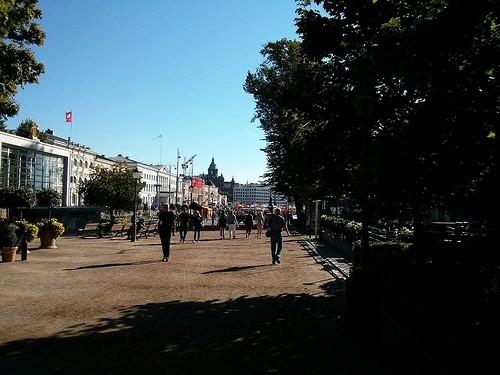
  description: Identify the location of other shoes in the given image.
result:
[180,238,182,242]
[221,237,223,240]
[248,236,250,238]
[183,241,185,243]
[192,240,195,243]
[273,254,280,264]
[232,235,236,239]
[197,240,199,243]
[246,234,247,238]
[257,235,261,239]
[229,235,231,239]
[223,235,225,238]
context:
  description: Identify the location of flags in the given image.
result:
[192,177,203,188]
[65,112,73,123]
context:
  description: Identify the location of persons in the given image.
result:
[244,210,253,240]
[267,208,290,264]
[211,213,216,226]
[256,211,265,240]
[153,203,175,261]
[96,216,115,238]
[127,218,145,239]
[218,209,229,239]
[178,208,190,243]
[189,210,204,243]
[228,210,239,239]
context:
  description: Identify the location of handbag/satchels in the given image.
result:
[200,223,204,227]
[266,231,271,237]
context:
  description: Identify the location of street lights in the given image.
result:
[131,166,142,242]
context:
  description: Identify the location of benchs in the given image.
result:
[77,220,158,241]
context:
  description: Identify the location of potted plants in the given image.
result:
[0,218,65,262]
